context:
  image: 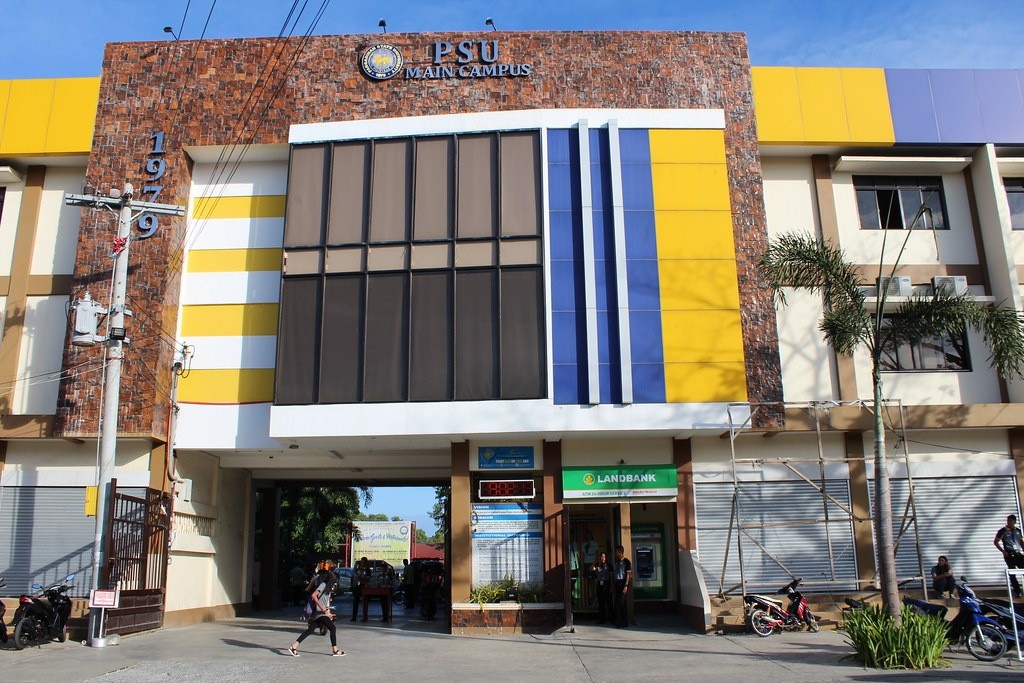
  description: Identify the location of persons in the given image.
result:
[350,557,371,624]
[589,552,616,625]
[304,568,332,620]
[288,571,348,657]
[994,514,1024,598]
[614,545,631,629]
[377,564,393,624]
[931,556,957,599]
[402,559,414,609]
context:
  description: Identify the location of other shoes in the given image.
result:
[949,594,956,599]
[610,622,616,625]
[360,617,368,622]
[380,618,388,622]
[938,594,946,598]
[350,618,356,622]
[597,620,606,624]
[1015,592,1022,598]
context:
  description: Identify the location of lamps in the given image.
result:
[163,27,177,40]
[378,18,386,34]
[485,16,497,31]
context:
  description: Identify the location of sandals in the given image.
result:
[333,650,347,656]
[287,645,300,657]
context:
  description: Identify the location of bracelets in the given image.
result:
[624,584,628,587]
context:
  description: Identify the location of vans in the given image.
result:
[352,559,398,595]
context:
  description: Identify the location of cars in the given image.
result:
[334,566,353,593]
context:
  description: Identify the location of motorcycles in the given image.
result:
[0,573,78,651]
[900,575,1024,661]
[741,579,819,637]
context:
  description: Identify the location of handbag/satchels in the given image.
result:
[306,596,314,615]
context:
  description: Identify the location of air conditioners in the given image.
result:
[930,275,969,299]
[875,276,912,297]
